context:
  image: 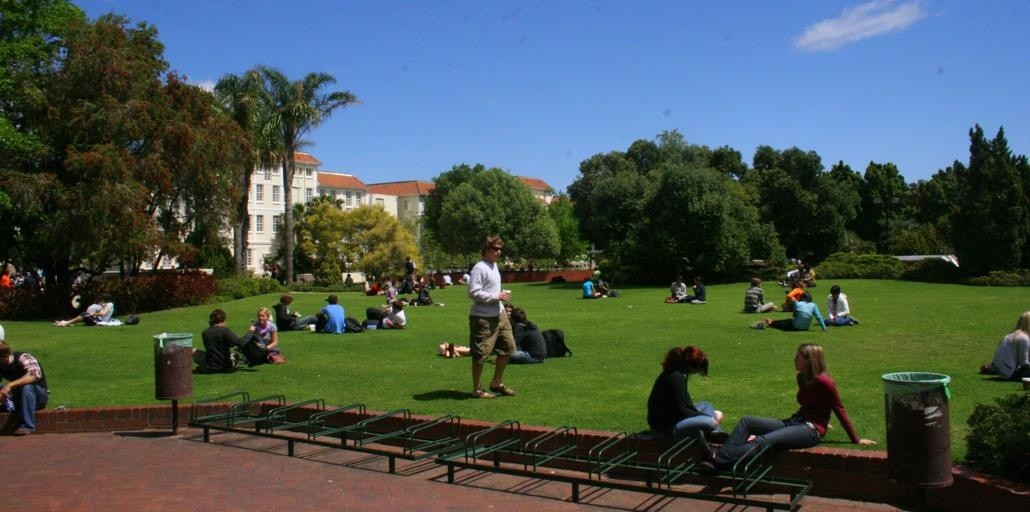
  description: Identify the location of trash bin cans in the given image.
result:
[881,372,954,494]
[152,332,193,436]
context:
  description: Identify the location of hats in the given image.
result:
[325,294,337,302]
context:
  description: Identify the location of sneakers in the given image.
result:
[445,343,459,359]
[849,315,860,325]
[693,430,728,473]
[14,427,32,435]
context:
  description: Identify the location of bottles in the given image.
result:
[5,399,15,412]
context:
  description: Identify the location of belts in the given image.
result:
[795,415,816,430]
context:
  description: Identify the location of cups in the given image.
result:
[503,290,511,295]
[1022,377,1030,389]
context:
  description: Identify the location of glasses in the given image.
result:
[490,246,502,252]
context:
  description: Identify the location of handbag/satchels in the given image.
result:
[124,315,139,325]
[345,317,362,332]
[543,329,572,358]
[241,336,266,365]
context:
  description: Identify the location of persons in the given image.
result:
[243,307,279,363]
[467,237,518,400]
[270,294,318,332]
[743,258,853,331]
[664,275,707,304]
[0,341,50,438]
[582,266,610,298]
[976,309,1030,388]
[647,346,725,465]
[347,256,433,329]
[442,270,471,286]
[190,306,257,374]
[695,340,878,471]
[440,302,546,363]
[55,293,115,327]
[316,293,346,334]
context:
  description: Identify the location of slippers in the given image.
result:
[473,384,515,398]
